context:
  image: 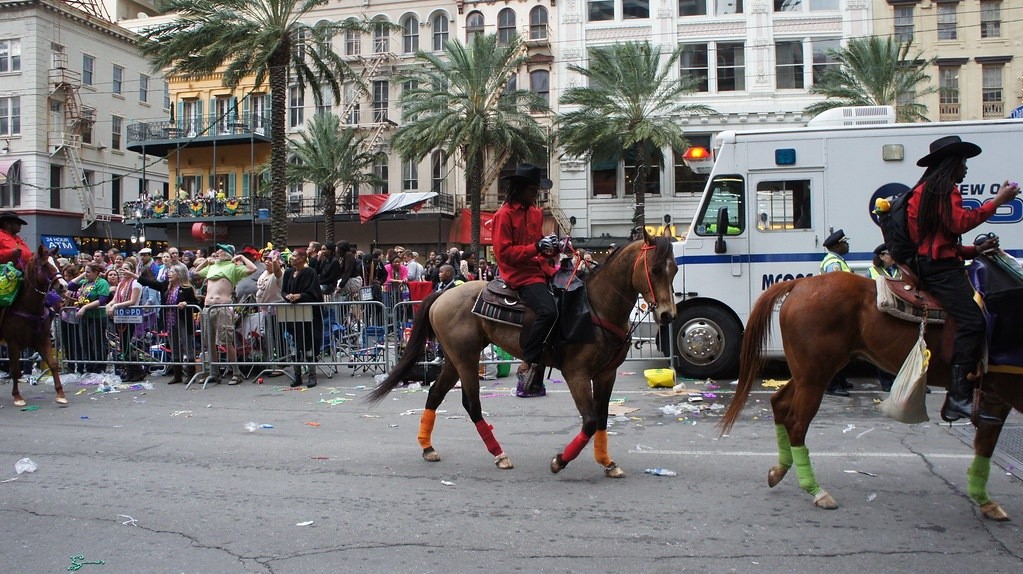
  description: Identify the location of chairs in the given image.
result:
[351,325,387,376]
[218,312,263,377]
[284,308,344,378]
[139,121,160,140]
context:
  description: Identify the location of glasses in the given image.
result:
[397,251,402,253]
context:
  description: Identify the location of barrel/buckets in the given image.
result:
[259,209,268,219]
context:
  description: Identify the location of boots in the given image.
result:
[168,359,182,384]
[184,359,196,383]
[517,363,546,396]
[291,360,302,387]
[942,347,1003,423]
[305,355,317,388]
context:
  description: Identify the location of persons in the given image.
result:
[205,187,216,215]
[0,212,33,272]
[308,240,423,355]
[50,246,288,385]
[820,230,853,395]
[608,243,617,255]
[863,243,930,394]
[425,247,504,380]
[282,248,323,388]
[492,163,598,398]
[882,134,1020,421]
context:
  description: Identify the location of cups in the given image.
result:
[673,383,685,393]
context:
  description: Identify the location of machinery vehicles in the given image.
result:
[656,105,1023,380]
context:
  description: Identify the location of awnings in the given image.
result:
[42,235,78,255]
[0,159,21,186]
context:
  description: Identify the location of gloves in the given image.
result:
[536,235,559,252]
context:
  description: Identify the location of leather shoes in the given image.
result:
[837,378,853,387]
[826,382,850,397]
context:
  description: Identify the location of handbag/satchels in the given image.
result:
[877,321,932,424]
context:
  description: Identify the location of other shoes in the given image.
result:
[120,371,285,385]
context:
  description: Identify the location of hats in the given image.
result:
[0,211,28,225]
[152,253,162,259]
[216,244,232,255]
[263,250,284,265]
[182,250,194,260]
[917,136,982,167]
[874,243,890,254]
[241,247,262,259]
[138,248,151,254]
[500,163,553,189]
[823,229,849,248]
[336,240,349,251]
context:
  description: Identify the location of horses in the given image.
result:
[368,225,680,478]
[0,239,69,406]
[715,270,1022,522]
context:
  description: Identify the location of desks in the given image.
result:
[163,127,182,139]
[228,123,248,134]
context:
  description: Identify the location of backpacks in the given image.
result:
[880,179,931,264]
